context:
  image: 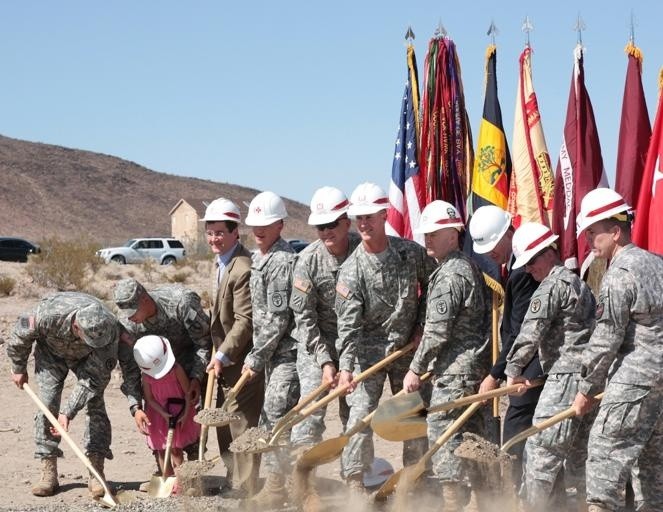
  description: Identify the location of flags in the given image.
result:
[614,42,652,213]
[385,41,427,251]
[505,46,556,229]
[471,43,513,213]
[551,44,610,284]
[631,66,663,259]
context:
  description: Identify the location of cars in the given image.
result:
[0,237,40,262]
[285,238,308,252]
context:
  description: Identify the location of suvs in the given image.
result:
[95,238,185,265]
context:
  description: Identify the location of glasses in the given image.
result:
[526,251,547,266]
[316,221,339,230]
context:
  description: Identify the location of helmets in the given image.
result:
[415,199,464,233]
[577,188,633,239]
[348,182,390,215]
[199,197,242,224]
[244,191,287,227]
[133,335,176,380]
[307,186,349,226]
[511,223,560,270]
[469,205,511,254]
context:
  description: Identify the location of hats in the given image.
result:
[76,303,115,348]
[114,278,143,318]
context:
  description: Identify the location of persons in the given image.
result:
[469,205,548,512]
[405,199,501,512]
[198,197,265,497]
[5,291,121,496]
[503,221,598,512]
[290,186,363,510]
[576,186,663,512]
[113,276,212,492]
[241,191,300,512]
[132,334,201,499]
[333,182,438,495]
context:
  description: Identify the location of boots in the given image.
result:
[88,452,112,496]
[33,457,59,496]
[442,482,459,512]
[347,476,369,499]
[253,472,287,504]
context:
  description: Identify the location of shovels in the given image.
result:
[297,372,434,471]
[230,376,341,453]
[258,342,416,452]
[9,368,137,510]
[148,398,187,499]
[454,392,606,462]
[375,401,483,503]
[179,345,215,476]
[193,368,252,427]
[369,379,545,442]
